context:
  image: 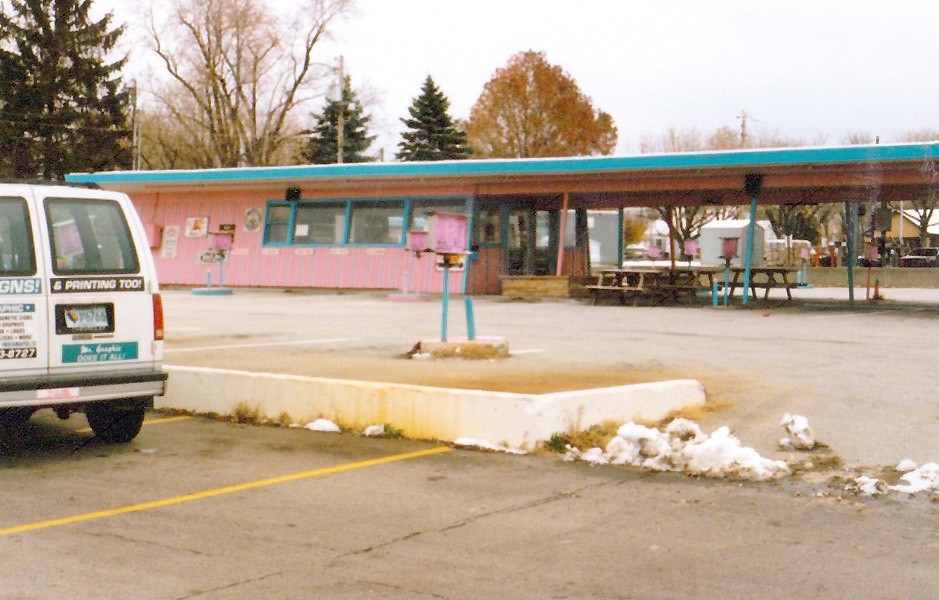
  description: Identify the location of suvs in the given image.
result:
[0,179,171,444]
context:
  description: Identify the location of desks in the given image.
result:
[596,267,799,307]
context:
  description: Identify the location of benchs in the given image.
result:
[586,281,808,307]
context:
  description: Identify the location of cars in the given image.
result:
[858,245,898,267]
[900,247,938,268]
